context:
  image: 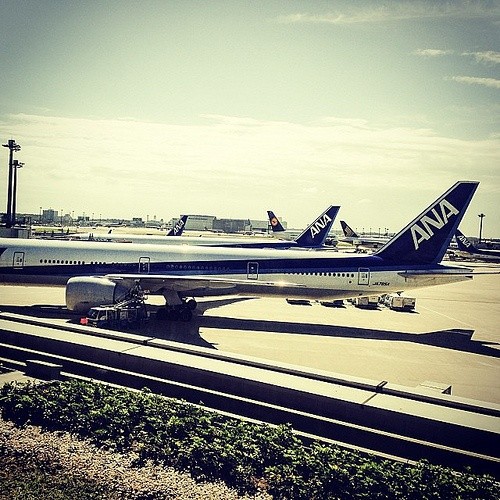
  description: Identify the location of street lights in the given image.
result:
[11,158,25,225]
[477,213,486,241]
[2,139,21,227]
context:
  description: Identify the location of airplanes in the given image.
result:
[0,179,482,324]
[242,209,500,264]
